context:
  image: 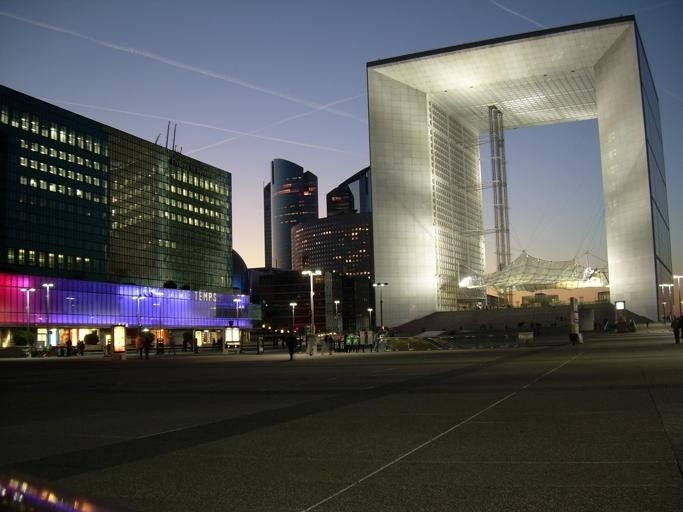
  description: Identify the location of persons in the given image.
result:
[343,332,352,354]
[135,333,144,358]
[670,315,681,345]
[285,329,297,360]
[78,341,84,356]
[328,334,332,355]
[351,332,359,353]
[65,337,71,356]
[167,332,175,360]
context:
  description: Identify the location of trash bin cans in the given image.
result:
[103,345,111,357]
[157,338,164,354]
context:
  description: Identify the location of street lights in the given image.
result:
[41,283,56,357]
[65,296,76,351]
[288,302,299,329]
[132,294,147,335]
[232,299,243,327]
[658,275,683,327]
[151,303,162,344]
[20,286,36,358]
[300,270,390,334]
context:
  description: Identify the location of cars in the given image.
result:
[251,328,283,342]
[0,470,138,511]
